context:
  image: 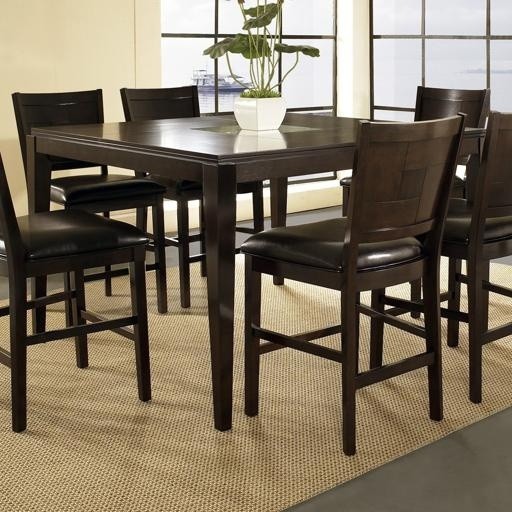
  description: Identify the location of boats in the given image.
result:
[190,68,256,93]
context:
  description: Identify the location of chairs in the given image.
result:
[240,113,467,455]
[340,86,492,217]
[11,89,168,327]
[386,112,512,404]
[2,159,150,434]
[120,86,264,309]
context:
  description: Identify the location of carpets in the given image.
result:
[1,252,512,511]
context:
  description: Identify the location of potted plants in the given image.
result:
[202,0,320,130]
